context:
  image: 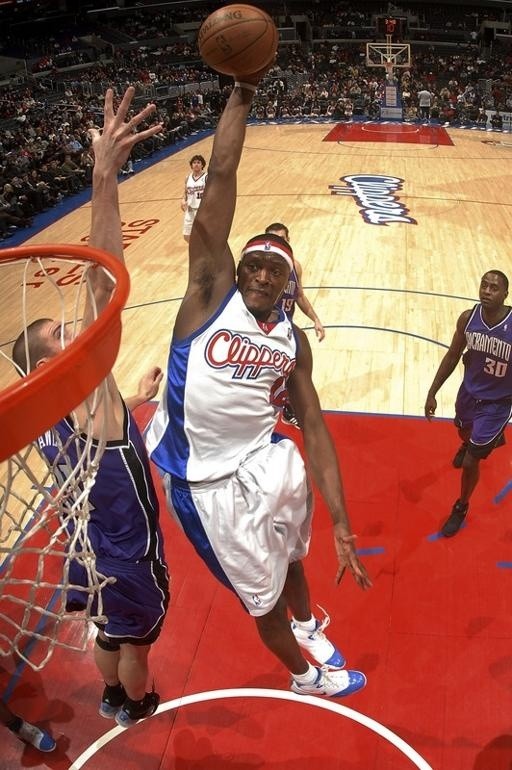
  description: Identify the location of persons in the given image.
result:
[424,269,512,538]
[1,702,59,753]
[265,222,326,433]
[10,81,173,729]
[140,61,374,700]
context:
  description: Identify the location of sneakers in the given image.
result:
[289,618,346,671]
[442,499,470,537]
[281,408,303,431]
[98,683,160,729]
[7,716,57,753]
[291,665,368,699]
[452,443,468,468]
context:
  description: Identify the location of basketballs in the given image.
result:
[198,4,279,75]
